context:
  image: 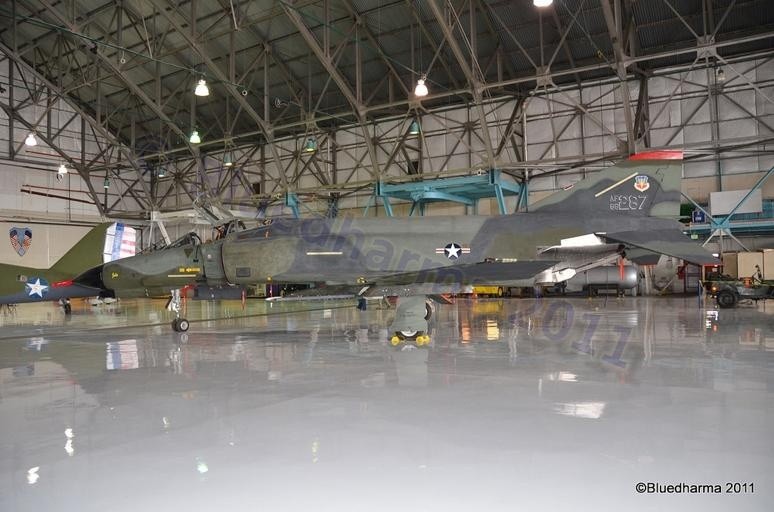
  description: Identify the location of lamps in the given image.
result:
[23,130,37,146]
[189,0,209,146]
[223,155,232,168]
[158,169,166,178]
[306,139,315,152]
[104,178,110,189]
[58,160,68,175]
[413,1,429,97]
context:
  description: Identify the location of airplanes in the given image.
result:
[68,149,724,344]
[0,218,122,312]
